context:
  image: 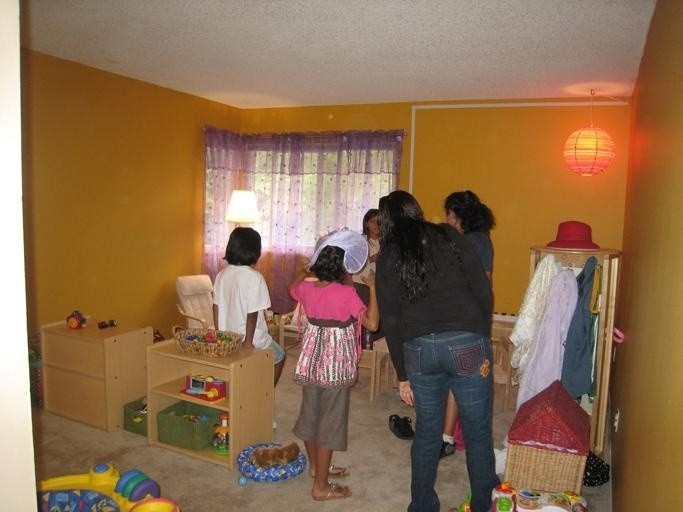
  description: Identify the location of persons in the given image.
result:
[375,190,503,512]
[290,227,380,501]
[352,209,379,350]
[439,190,497,459]
[214,227,287,429]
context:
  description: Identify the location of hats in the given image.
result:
[546,221,601,249]
[309,229,369,274]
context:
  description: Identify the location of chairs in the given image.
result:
[176,274,214,331]
[280,310,303,351]
[357,348,390,399]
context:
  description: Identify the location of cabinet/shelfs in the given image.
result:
[146,336,275,469]
[40,315,153,432]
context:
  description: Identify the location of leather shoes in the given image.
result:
[389,414,415,440]
[440,441,456,458]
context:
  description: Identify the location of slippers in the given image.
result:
[311,464,350,478]
[313,481,352,501]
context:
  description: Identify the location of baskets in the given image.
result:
[159,403,221,451]
[124,396,148,436]
[172,325,244,358]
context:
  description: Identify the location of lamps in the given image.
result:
[563,89,617,175]
[223,190,260,228]
